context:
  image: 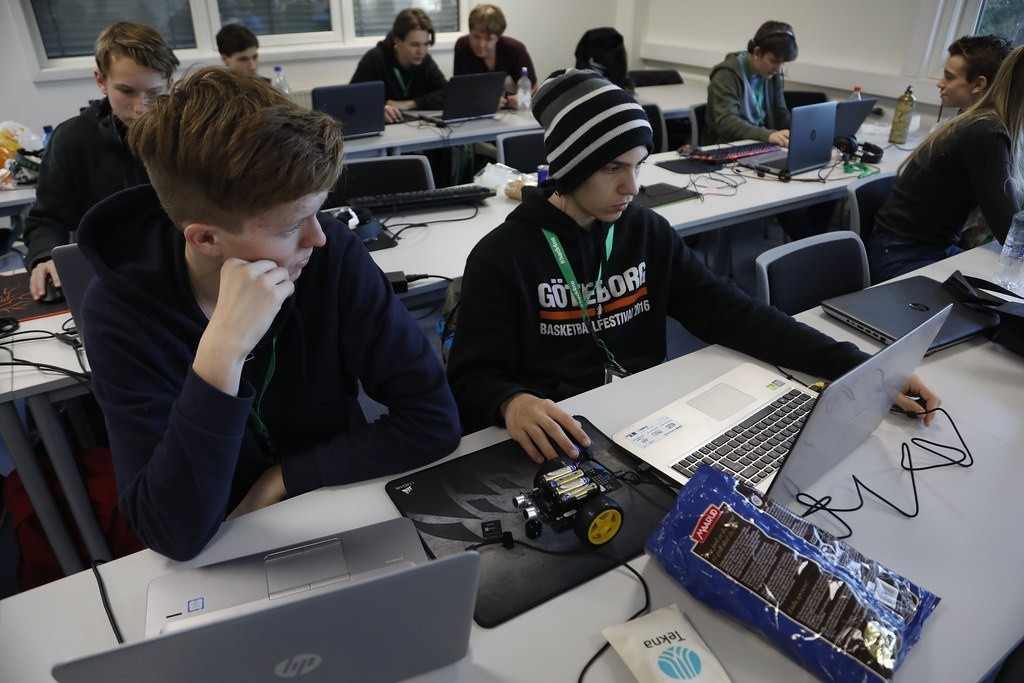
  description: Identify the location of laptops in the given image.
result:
[52,515,481,683]
[311,80,385,141]
[835,98,879,142]
[401,70,506,122]
[53,242,96,347]
[612,301,954,505]
[820,275,1001,357]
[737,100,837,177]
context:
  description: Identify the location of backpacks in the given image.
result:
[574,27,634,96]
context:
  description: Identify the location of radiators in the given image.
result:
[289,87,313,111]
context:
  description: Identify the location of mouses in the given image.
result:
[38,274,66,304]
[386,115,404,125]
[537,424,597,461]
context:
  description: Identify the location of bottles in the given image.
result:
[992,209,1024,291]
[848,86,862,100]
[43,125,54,147]
[517,68,533,113]
[271,66,291,96]
[888,85,916,144]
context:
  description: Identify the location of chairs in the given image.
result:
[495,129,549,174]
[783,90,827,113]
[754,230,869,316]
[319,155,436,211]
[846,170,904,245]
[640,103,668,155]
[628,68,692,151]
[687,102,708,147]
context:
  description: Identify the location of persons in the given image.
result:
[864,46,1024,284]
[446,68,942,465]
[701,21,798,147]
[936,34,1017,248]
[24,20,181,301]
[216,24,259,77]
[75,62,461,561]
[350,5,635,190]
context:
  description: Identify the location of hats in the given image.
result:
[531,68,653,192]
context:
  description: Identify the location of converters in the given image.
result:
[385,270,410,294]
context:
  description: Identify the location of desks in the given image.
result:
[0,73,1024,683]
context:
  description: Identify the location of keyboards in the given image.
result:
[344,185,497,218]
[688,142,781,161]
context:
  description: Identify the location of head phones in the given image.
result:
[836,135,884,165]
[747,30,795,54]
